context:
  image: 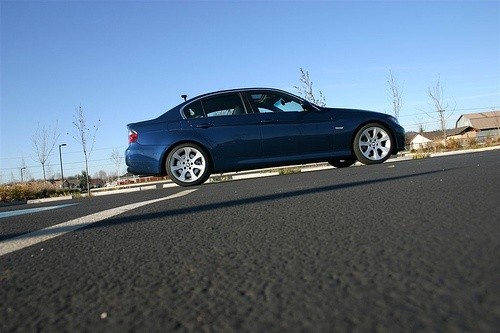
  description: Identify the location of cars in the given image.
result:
[125,88,406,187]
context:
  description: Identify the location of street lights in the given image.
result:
[21,168,26,182]
[59,144,67,189]
